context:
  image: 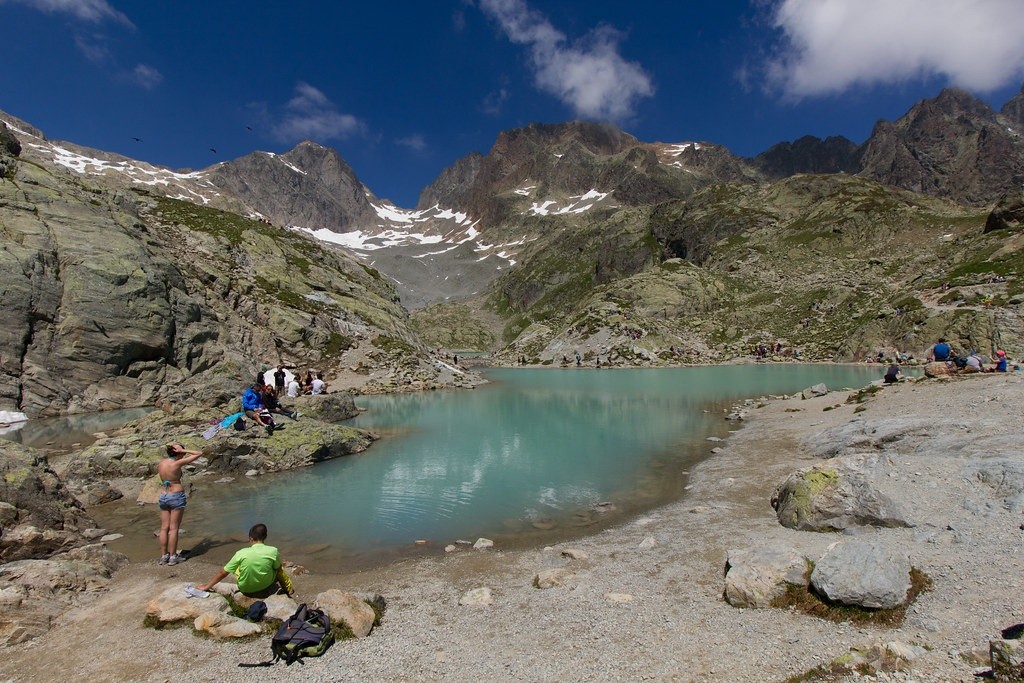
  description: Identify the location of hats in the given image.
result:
[247,601,267,621]
[996,351,1004,357]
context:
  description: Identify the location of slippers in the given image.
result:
[184,585,210,599]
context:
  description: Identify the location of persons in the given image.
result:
[158,445,203,566]
[198,524,295,595]
[242,365,327,435]
[934,338,1007,374]
[749,342,799,358]
[884,364,899,383]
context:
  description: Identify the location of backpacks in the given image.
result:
[270,603,333,666]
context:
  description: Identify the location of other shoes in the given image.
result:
[169,556,186,565]
[266,425,272,431]
[273,422,285,429]
[291,412,297,418]
[297,413,304,417]
[160,553,170,565]
